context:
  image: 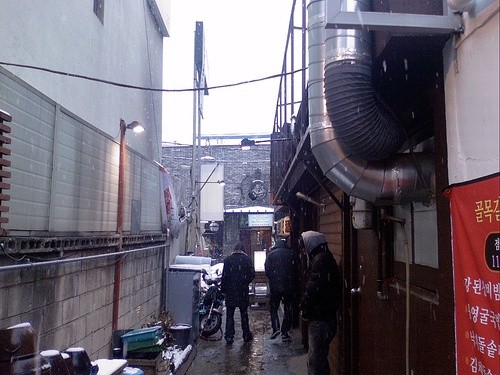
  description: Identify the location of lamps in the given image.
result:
[194,179,226,186]
[240,138,291,151]
[248,190,276,201]
[125,121,145,133]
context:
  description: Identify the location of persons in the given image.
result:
[221,241,255,343]
[265,239,296,341]
[301,231,342,375]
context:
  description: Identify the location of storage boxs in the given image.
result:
[112,325,170,375]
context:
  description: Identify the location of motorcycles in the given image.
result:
[198,273,223,337]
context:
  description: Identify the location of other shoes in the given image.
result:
[226,338,235,344]
[270,330,281,340]
[243,335,253,342]
[281,334,291,341]
